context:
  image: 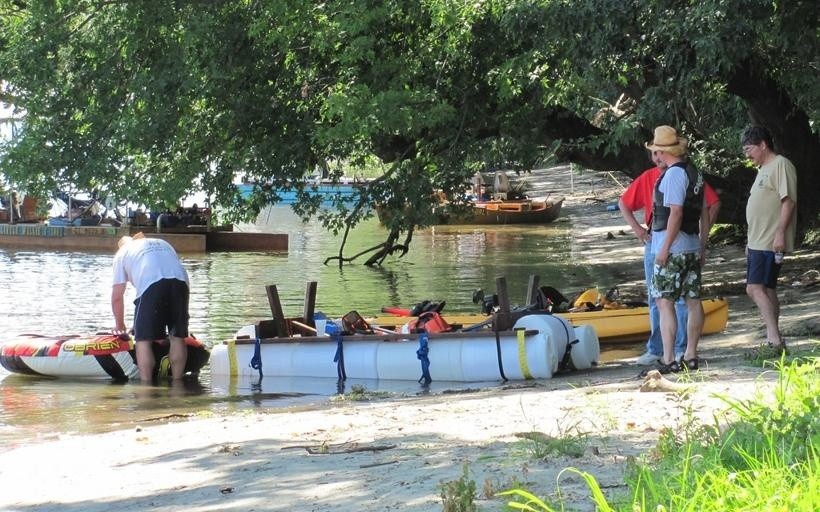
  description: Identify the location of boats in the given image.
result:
[363,284,729,342]
[0,332,210,379]
[210,273,600,392]
[373,189,566,226]
[236,176,395,200]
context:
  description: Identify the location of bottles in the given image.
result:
[394,323,410,342]
[525,195,528,200]
[775,249,784,264]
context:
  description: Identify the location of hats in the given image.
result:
[643,125,688,157]
[114,231,145,253]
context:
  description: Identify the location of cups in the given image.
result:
[315,319,326,336]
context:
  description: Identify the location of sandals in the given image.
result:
[679,356,698,370]
[639,360,680,378]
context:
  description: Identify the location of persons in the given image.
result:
[110,231,191,382]
[739,124,799,362]
[637,125,710,377]
[618,140,722,367]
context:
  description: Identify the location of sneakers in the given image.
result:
[744,340,787,360]
[635,352,684,365]
